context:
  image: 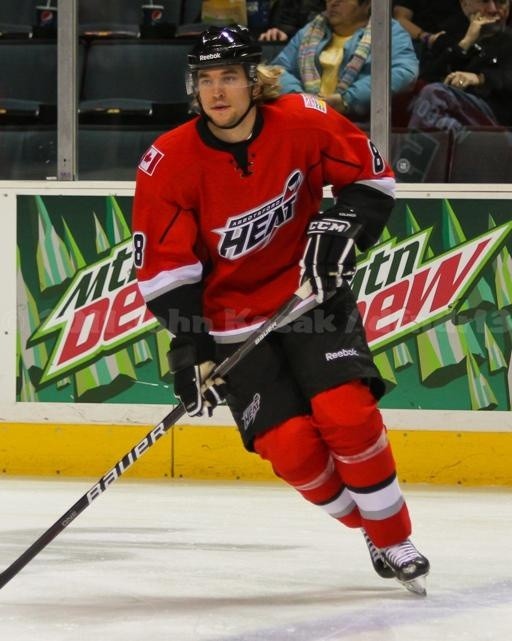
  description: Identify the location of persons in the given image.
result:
[132,22,429,581]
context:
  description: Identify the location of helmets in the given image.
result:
[183,23,264,100]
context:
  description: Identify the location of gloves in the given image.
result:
[298,205,370,304]
[166,330,233,418]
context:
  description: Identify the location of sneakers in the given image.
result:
[361,526,394,578]
[380,538,430,582]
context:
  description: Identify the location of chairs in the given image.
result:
[359,121,512,183]
[1,1,203,183]
